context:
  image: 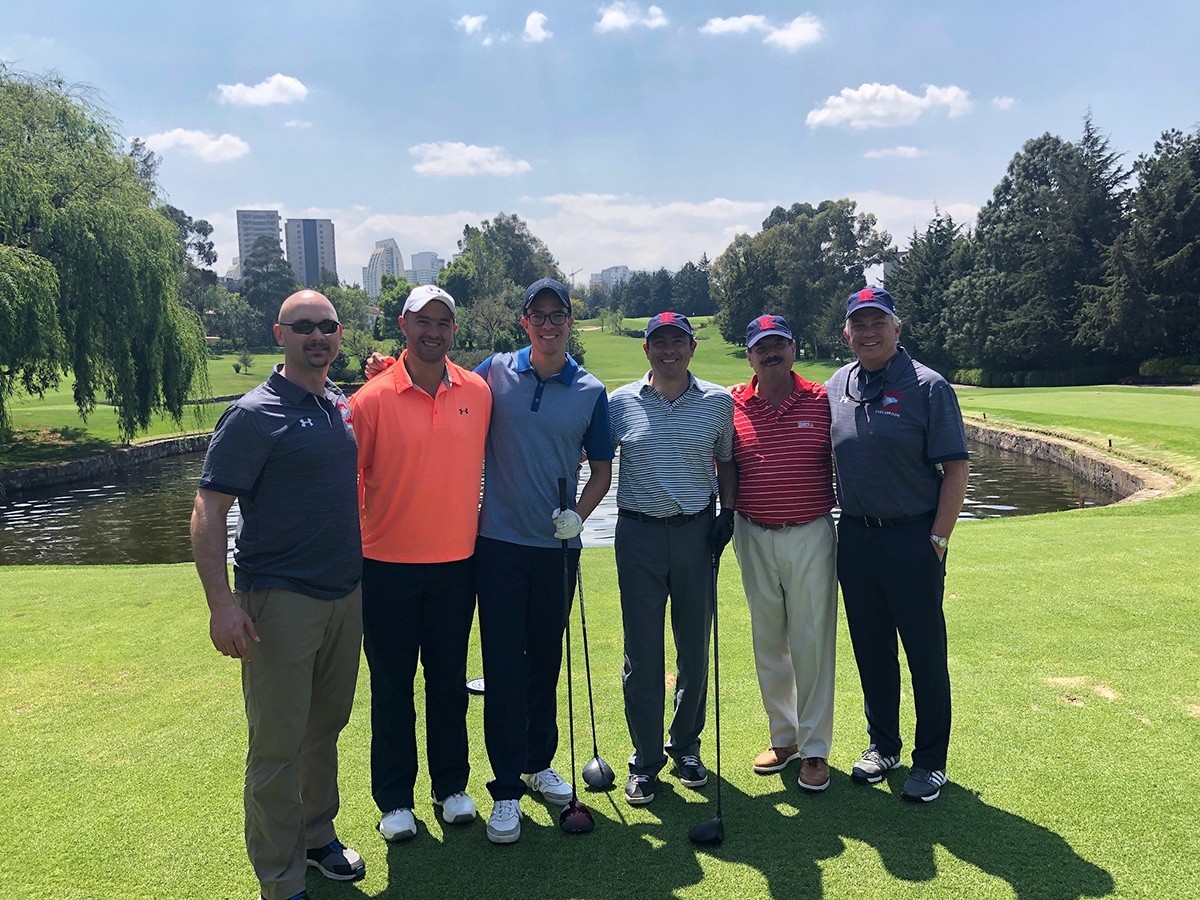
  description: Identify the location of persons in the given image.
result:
[715,313,838,792]
[347,284,493,841]
[581,311,736,804]
[190,291,366,900]
[823,287,969,805]
[359,278,614,844]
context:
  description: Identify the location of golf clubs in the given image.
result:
[686,492,727,848]
[559,477,596,832]
[581,561,618,785]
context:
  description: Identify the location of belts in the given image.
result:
[618,502,711,526]
[737,510,807,530]
[848,512,934,528]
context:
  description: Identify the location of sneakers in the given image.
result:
[625,774,657,804]
[798,757,830,790]
[851,749,901,784]
[260,891,311,900]
[753,744,801,773]
[380,809,417,841]
[901,764,947,802]
[307,836,365,881]
[431,790,476,824]
[487,799,523,843]
[520,768,578,806]
[675,755,708,787]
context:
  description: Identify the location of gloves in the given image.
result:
[707,508,735,550]
[552,508,584,540]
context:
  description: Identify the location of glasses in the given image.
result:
[844,369,886,404]
[279,320,339,334]
[526,312,569,326]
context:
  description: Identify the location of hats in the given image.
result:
[745,315,792,349]
[402,285,456,319]
[845,288,895,320]
[523,278,570,315]
[646,312,693,340]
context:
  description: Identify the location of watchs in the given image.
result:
[928,532,950,549]
